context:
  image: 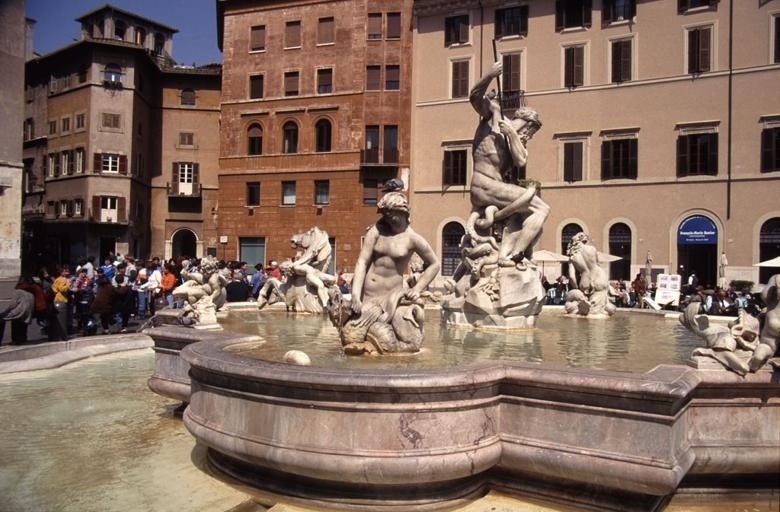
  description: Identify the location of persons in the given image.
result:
[15,250,282,340]
[608,264,764,316]
[748,273,779,372]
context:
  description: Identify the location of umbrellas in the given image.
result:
[751,255,780,267]
